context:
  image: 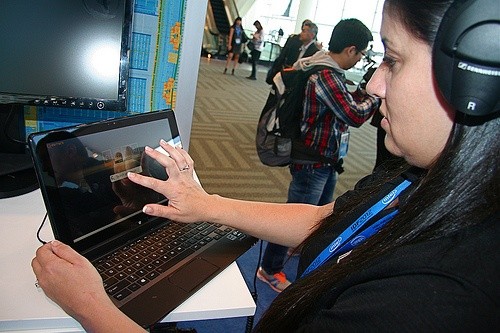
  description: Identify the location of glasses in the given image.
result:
[356,47,367,60]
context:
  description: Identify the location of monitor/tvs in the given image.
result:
[0,0,136,199]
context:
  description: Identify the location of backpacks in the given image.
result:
[256,65,347,167]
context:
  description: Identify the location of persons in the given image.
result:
[30,0,500,333]
[268,19,325,68]
[257,19,382,293]
[224,17,243,75]
[246,21,264,80]
[363,45,376,71]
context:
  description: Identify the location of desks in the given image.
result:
[0,163,256,333]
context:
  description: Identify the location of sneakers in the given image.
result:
[257,266,291,293]
[287,247,302,257]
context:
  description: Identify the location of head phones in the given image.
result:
[432,0,500,115]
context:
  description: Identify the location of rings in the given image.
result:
[180,166,189,171]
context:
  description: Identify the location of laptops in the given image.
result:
[27,108,259,329]
